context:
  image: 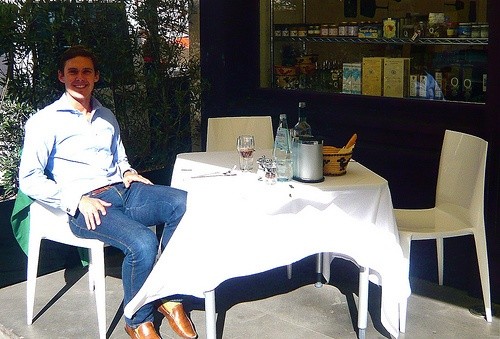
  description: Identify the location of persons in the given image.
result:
[19,46,197,339]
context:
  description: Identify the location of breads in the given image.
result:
[344,133,357,148]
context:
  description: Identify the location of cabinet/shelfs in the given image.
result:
[271,36,488,105]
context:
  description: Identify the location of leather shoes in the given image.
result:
[124,321,161,339]
[154,301,198,339]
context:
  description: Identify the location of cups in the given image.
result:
[237,135,255,172]
[292,137,325,183]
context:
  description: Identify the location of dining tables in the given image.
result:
[176,152,389,339]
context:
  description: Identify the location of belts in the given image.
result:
[86,185,114,197]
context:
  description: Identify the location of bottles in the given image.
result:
[409,52,479,100]
[293,101,311,138]
[273,113,293,182]
[313,57,343,91]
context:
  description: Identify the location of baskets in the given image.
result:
[322,146,353,176]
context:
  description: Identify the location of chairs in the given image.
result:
[206,116,274,150]
[26,197,106,339]
[393,130,492,332]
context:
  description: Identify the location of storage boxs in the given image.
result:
[341,57,408,97]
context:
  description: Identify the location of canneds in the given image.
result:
[417,20,488,39]
[274,22,358,38]
